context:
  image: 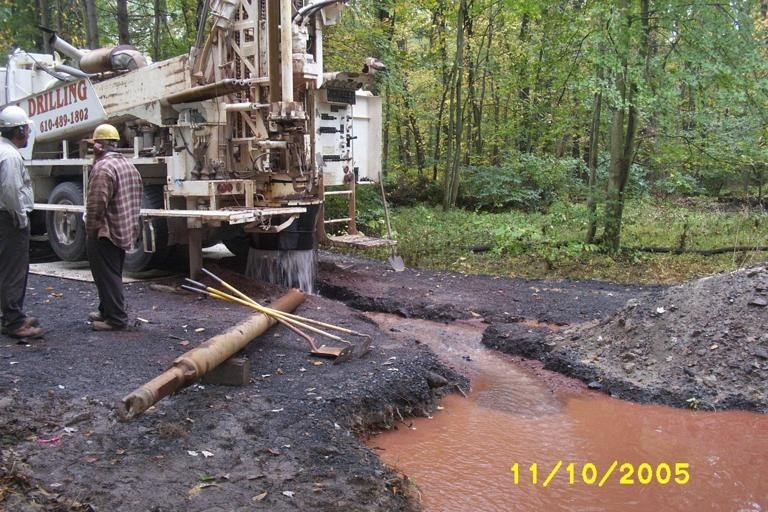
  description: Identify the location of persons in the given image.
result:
[81,123,145,332]
[342,165,354,184]
[0,105,46,339]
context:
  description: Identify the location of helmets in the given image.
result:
[92,124,120,141]
[0,105,34,128]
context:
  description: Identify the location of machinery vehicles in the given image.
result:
[0,0,397,286]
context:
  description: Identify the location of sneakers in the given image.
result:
[0,314,37,334]
[90,317,128,331]
[11,327,47,338]
[86,306,105,322]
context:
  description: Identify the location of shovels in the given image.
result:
[201,267,346,356]
[378,171,405,273]
